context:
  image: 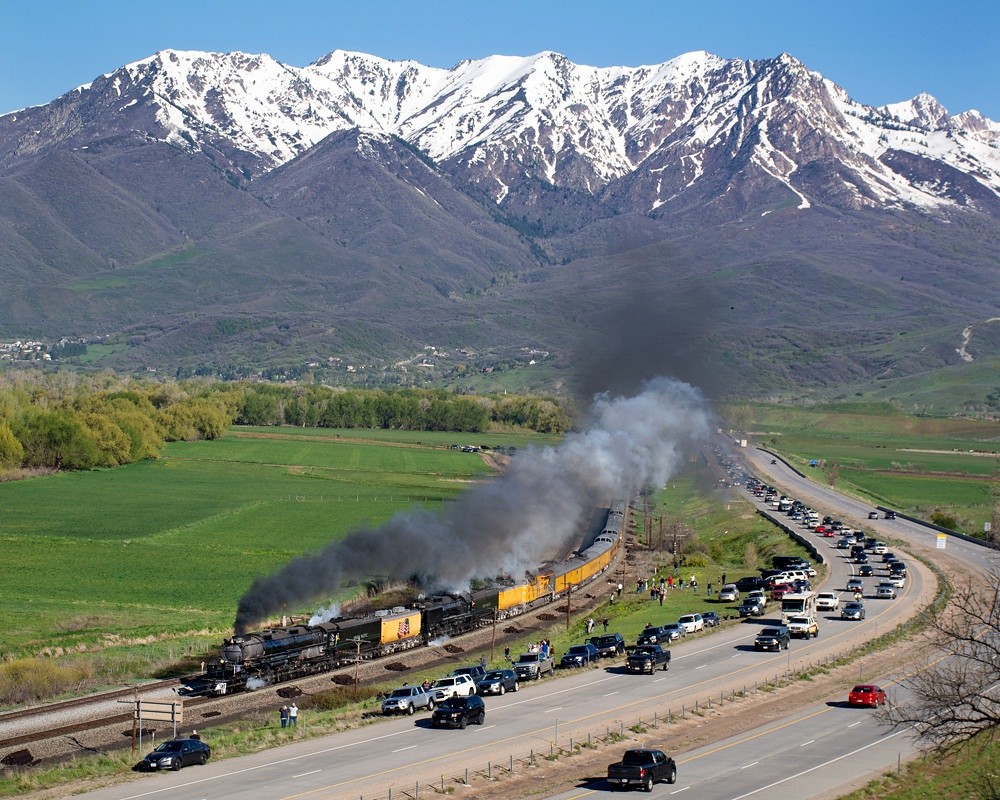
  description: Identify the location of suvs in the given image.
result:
[786,618,819,640]
[561,644,600,669]
[431,694,484,730]
[447,665,487,694]
[427,673,477,703]
[584,633,625,658]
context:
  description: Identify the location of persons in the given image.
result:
[603,616,609,631]
[402,679,438,692]
[679,578,683,590]
[610,582,622,606]
[505,645,509,657]
[288,702,299,727]
[175,732,186,739]
[650,577,667,606]
[278,705,290,728]
[637,578,643,593]
[528,637,555,656]
[668,576,673,591]
[691,574,697,592]
[706,581,711,596]
[479,654,487,670]
[190,730,200,740]
[718,572,726,585]
[674,561,679,574]
[854,585,864,603]
[587,617,594,633]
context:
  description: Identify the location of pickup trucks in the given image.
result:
[515,651,556,682]
[625,644,671,675]
[607,748,678,792]
[381,684,436,717]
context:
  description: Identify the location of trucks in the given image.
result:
[780,592,817,625]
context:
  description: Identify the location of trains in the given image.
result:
[197,497,626,698]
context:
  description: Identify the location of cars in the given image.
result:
[143,739,210,772]
[636,611,721,646]
[451,444,504,453]
[849,684,887,709]
[705,442,907,622]
[475,668,520,696]
[754,625,791,653]
